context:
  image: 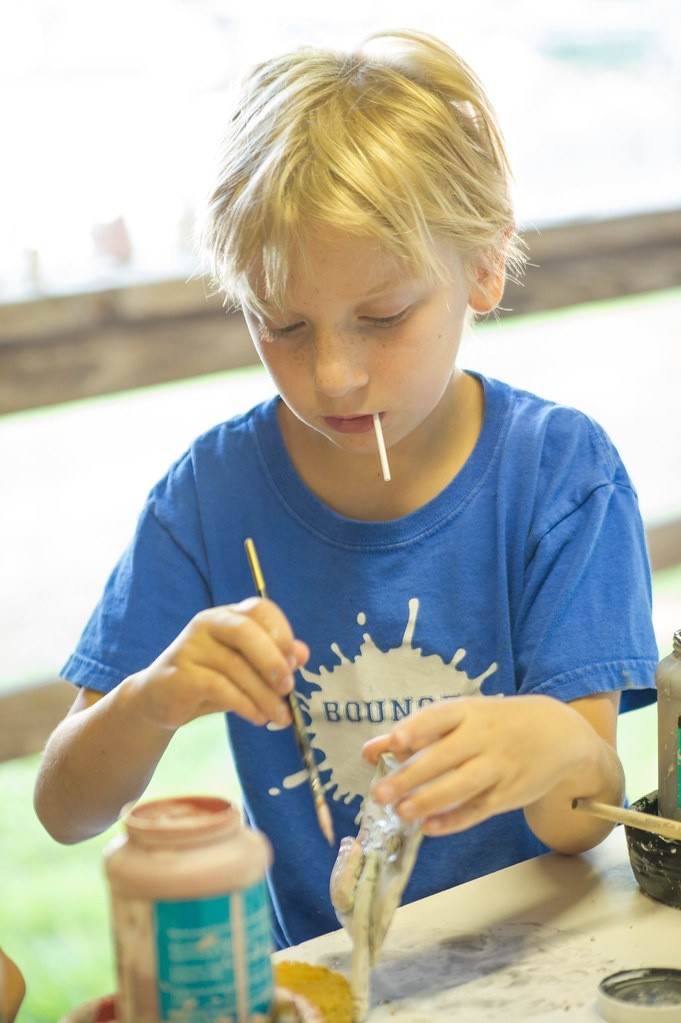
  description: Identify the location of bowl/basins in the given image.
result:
[626,789,681,912]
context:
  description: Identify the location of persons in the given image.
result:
[32,27,662,949]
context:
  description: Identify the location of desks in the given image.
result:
[104,774,679,1022]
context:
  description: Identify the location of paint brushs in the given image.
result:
[241,537,336,849]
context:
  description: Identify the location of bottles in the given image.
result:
[105,793,279,1023]
